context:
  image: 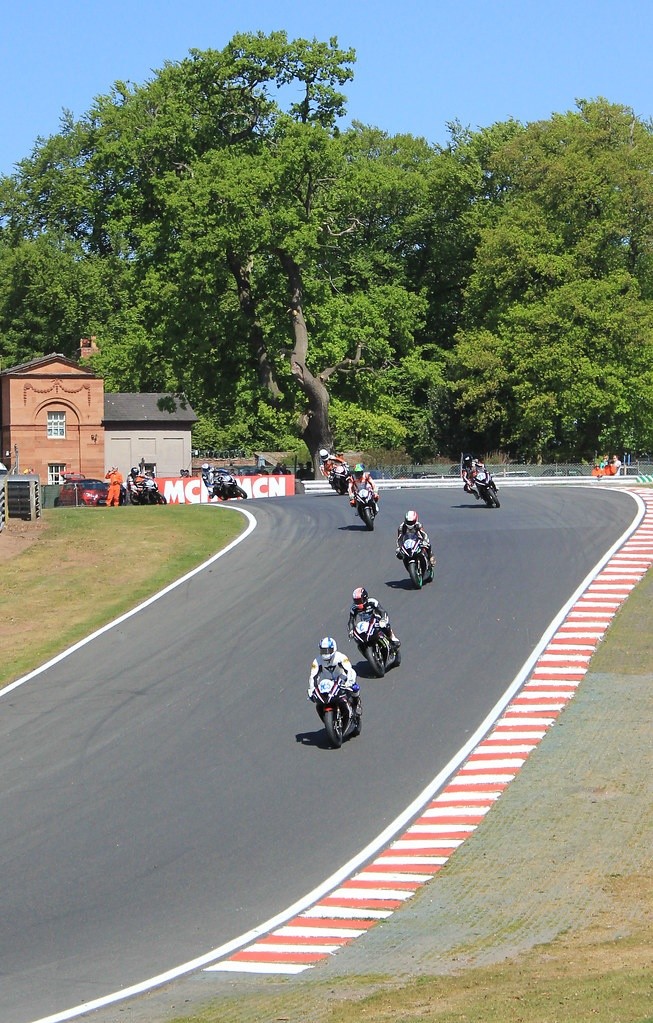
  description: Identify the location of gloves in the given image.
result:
[422,540,428,546]
[348,629,354,639]
[379,619,388,628]
[344,681,353,688]
[396,547,401,555]
[308,687,316,698]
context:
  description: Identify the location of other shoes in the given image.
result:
[353,701,362,716]
[389,633,401,647]
[428,555,435,565]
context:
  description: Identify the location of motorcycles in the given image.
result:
[212,472,248,500]
[329,463,349,495]
[354,482,380,532]
[130,471,167,505]
[351,612,402,678]
[397,532,435,589]
[310,671,363,749]
[470,468,501,508]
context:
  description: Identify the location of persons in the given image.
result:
[396,510,436,567]
[106,466,123,507]
[296,461,315,480]
[179,469,191,478]
[258,466,269,476]
[307,636,362,716]
[272,462,291,475]
[126,468,148,497]
[347,587,401,648]
[319,449,349,479]
[348,463,380,512]
[462,456,498,500]
[201,463,230,499]
[593,455,621,476]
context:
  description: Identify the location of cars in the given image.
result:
[391,463,643,479]
[54,471,126,507]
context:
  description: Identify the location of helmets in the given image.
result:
[201,463,211,474]
[318,635,337,662]
[403,510,418,530]
[131,466,139,478]
[463,454,473,467]
[353,588,369,611]
[353,463,366,479]
[319,449,330,462]
